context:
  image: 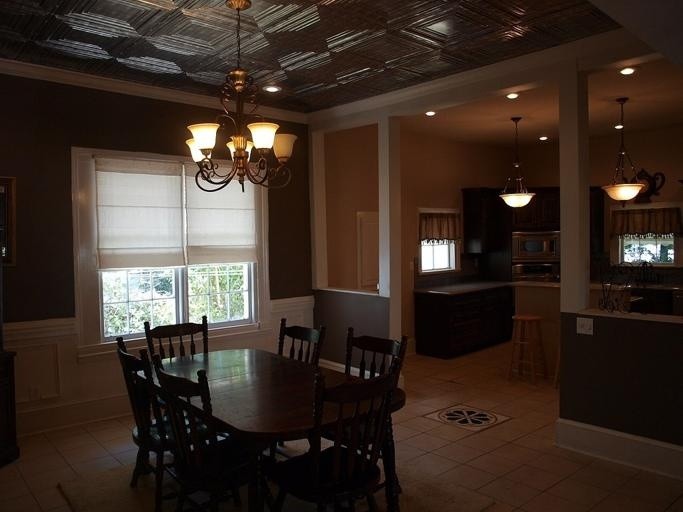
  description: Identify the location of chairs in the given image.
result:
[251,355,401,511]
[144,315,208,415]
[116,336,192,511]
[320,327,408,512]
[275,319,327,447]
[151,352,272,512]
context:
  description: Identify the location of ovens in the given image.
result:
[511,231,560,282]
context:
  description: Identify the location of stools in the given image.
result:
[508,314,549,385]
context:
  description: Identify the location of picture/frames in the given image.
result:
[0,176,17,268]
[356,211,378,290]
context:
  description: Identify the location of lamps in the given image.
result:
[601,97,645,208]
[185,0,298,191]
[498,117,537,208]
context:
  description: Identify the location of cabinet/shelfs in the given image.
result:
[512,187,561,231]
[413,281,512,359]
[462,187,511,255]
[590,185,604,260]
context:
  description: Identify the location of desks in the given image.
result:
[136,347,406,511]
